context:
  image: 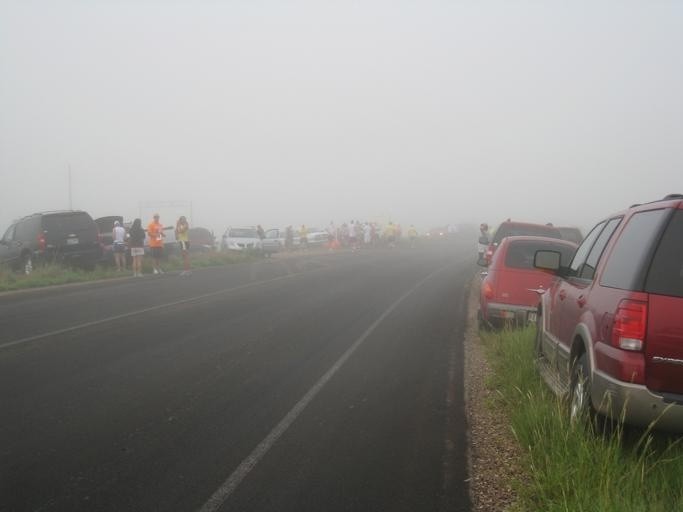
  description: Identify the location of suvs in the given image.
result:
[532,194,682,428]
[477,220,584,264]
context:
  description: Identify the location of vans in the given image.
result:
[1,211,101,276]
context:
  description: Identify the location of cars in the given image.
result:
[190,226,330,250]
[94,215,131,262]
[480,235,579,325]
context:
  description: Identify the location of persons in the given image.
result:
[111,220,126,272]
[254,219,493,253]
[174,215,191,277]
[128,217,145,279]
[147,213,166,275]
[477,222,489,266]
